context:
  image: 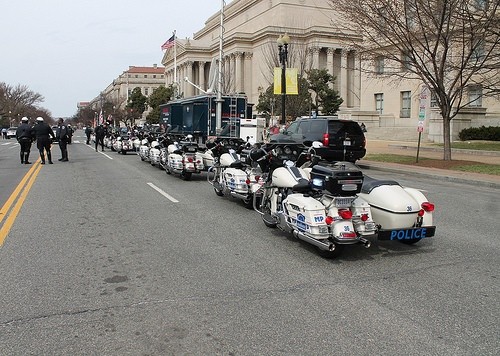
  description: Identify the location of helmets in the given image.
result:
[36,117,43,121]
[21,117,28,120]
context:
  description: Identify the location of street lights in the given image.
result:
[277,33,290,124]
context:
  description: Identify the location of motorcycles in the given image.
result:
[249,137,436,258]
[91,124,215,181]
[205,136,263,210]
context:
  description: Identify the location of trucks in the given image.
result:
[180,94,248,143]
[159,102,181,128]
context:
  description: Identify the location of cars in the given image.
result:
[48,126,69,143]
[6,126,20,139]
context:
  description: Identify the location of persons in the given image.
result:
[32,117,56,164]
[56,118,68,162]
[85,124,92,145]
[31,123,36,144]
[67,124,74,144]
[2,126,7,139]
[94,123,106,152]
[16,117,33,164]
[106,121,166,139]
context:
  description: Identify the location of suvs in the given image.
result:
[270,117,367,164]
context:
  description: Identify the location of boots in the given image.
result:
[24,152,31,164]
[20,151,25,164]
[40,151,45,164]
[47,151,53,164]
[58,149,68,162]
